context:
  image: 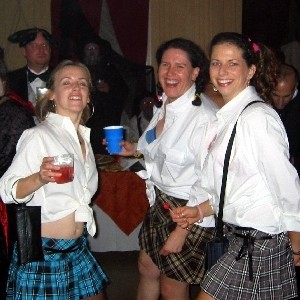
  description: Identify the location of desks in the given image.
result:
[85,155,149,253]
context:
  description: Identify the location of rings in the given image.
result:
[180,211,185,217]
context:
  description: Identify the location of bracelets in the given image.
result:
[195,204,203,223]
[184,226,192,232]
[133,143,141,158]
[293,251,300,255]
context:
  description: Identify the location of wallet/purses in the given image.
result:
[14,204,45,265]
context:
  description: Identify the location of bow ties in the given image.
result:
[28,70,51,82]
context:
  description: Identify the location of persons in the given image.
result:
[0,59,111,300]
[0,28,300,300]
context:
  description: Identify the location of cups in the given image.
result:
[103,126,124,155]
[37,87,48,103]
[50,153,74,184]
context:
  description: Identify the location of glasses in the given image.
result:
[26,43,51,50]
